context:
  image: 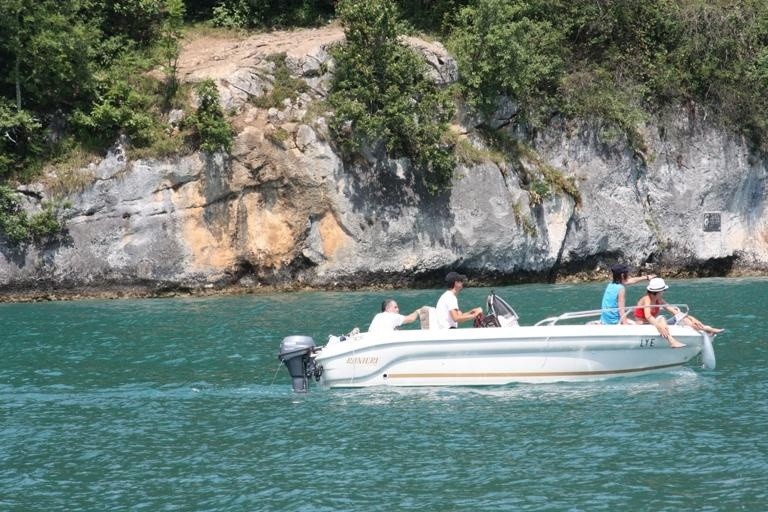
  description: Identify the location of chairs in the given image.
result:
[419,305,437,330]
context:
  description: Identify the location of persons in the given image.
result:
[600,264,635,325]
[436,272,483,329]
[368,300,428,333]
[633,278,725,348]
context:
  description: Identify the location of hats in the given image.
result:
[611,264,633,275]
[444,272,467,283]
[646,278,669,292]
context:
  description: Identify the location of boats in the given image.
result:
[278,300,719,396]
